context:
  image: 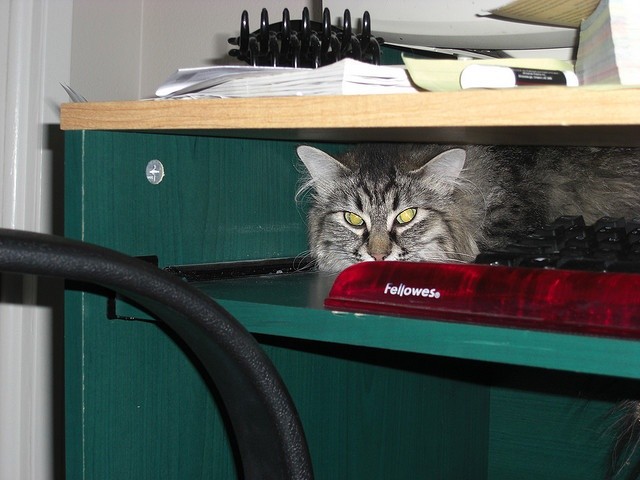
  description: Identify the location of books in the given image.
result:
[575,0,636,86]
[200,57,417,99]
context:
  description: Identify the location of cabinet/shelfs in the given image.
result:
[59,86,640,479]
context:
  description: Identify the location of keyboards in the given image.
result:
[324,214,640,341]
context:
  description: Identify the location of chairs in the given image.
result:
[0,229,315,480]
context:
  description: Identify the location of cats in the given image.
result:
[294,140,640,277]
